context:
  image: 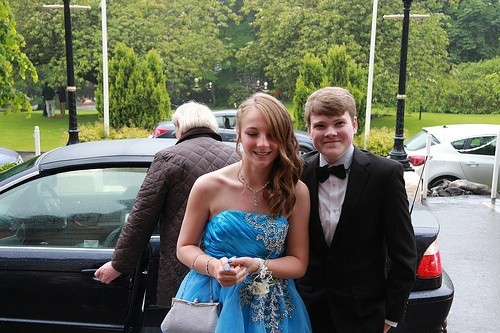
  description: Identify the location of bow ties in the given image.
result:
[312,164,346,184]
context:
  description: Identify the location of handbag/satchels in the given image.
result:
[161,297,220,333]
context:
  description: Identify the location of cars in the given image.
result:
[387,124,500,197]
[148,109,317,160]
[0,147,24,171]
[0,138,455,333]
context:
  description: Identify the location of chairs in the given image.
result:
[104,209,129,248]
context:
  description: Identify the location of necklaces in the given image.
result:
[238,162,270,207]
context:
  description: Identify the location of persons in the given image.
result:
[167,55,312,333]
[298,87,417,333]
[95,100,244,308]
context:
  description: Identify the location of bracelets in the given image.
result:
[206,257,215,276]
[252,259,265,276]
